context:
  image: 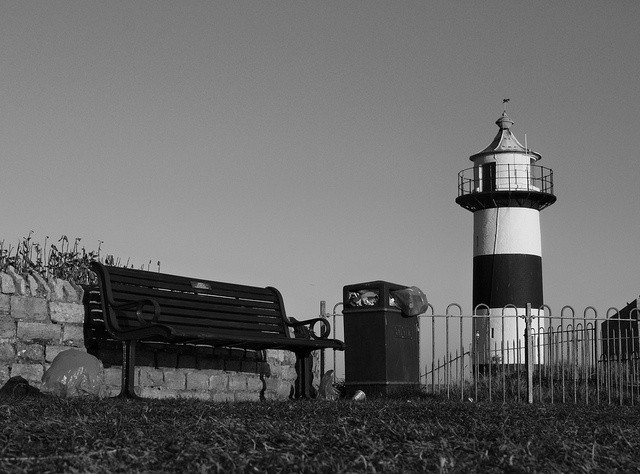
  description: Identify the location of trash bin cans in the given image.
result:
[342,280,420,399]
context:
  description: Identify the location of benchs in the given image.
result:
[88,259,347,402]
